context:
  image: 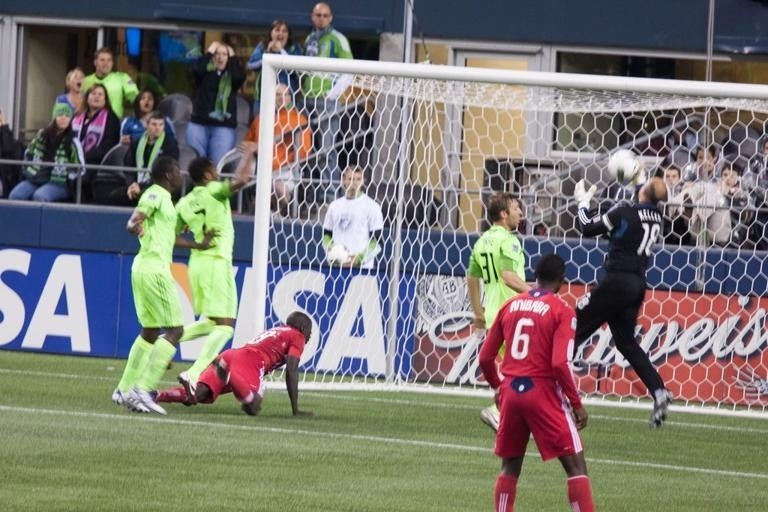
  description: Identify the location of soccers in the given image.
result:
[608,150,639,184]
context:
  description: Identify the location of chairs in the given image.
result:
[550,127,767,240]
[18,96,304,216]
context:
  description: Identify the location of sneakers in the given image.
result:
[213,355,232,385]
[150,388,161,402]
[650,389,673,428]
[130,387,167,415]
[177,371,198,405]
[111,389,151,413]
[480,407,499,431]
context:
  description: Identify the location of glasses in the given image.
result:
[313,11,329,18]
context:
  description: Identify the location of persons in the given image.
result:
[478,253,595,511]
[567,175,672,430]
[592,122,768,253]
[468,192,535,440]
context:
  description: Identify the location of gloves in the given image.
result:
[573,178,598,210]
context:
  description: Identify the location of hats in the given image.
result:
[52,103,74,118]
